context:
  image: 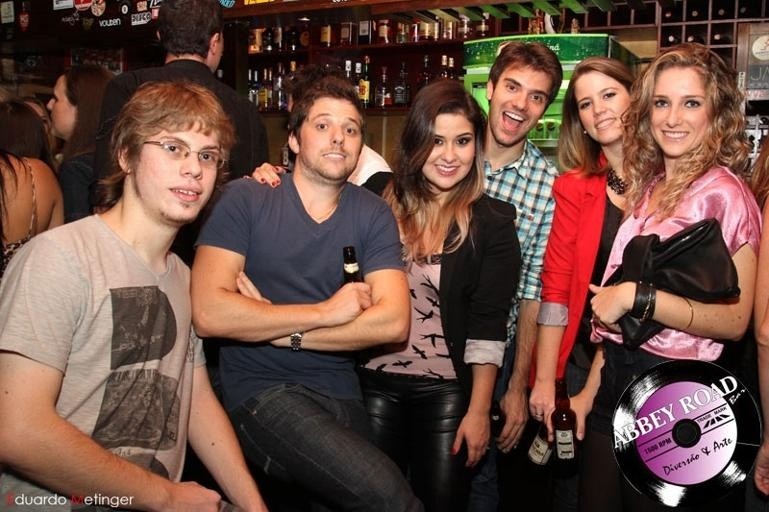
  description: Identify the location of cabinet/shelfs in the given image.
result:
[657,0,769,181]
[227,0,497,119]
[497,0,658,35]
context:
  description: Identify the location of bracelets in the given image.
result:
[680,295,695,336]
[633,277,659,323]
[289,330,305,354]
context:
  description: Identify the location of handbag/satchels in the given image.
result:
[600,217,740,352]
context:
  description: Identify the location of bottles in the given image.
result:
[525,422,555,466]
[660,3,757,46]
[244,16,489,54]
[551,378,578,458]
[246,57,456,108]
[488,399,516,453]
[342,248,363,285]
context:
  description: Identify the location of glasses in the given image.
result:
[143,140,227,170]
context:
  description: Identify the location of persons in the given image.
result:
[479,41,564,510]
[239,76,522,511]
[531,53,642,511]
[190,74,430,512]
[750,126,769,511]
[567,45,759,512]
[0,98,66,278]
[0,80,272,512]
[100,1,269,182]
[45,61,114,226]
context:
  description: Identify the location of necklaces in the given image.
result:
[603,160,632,197]
